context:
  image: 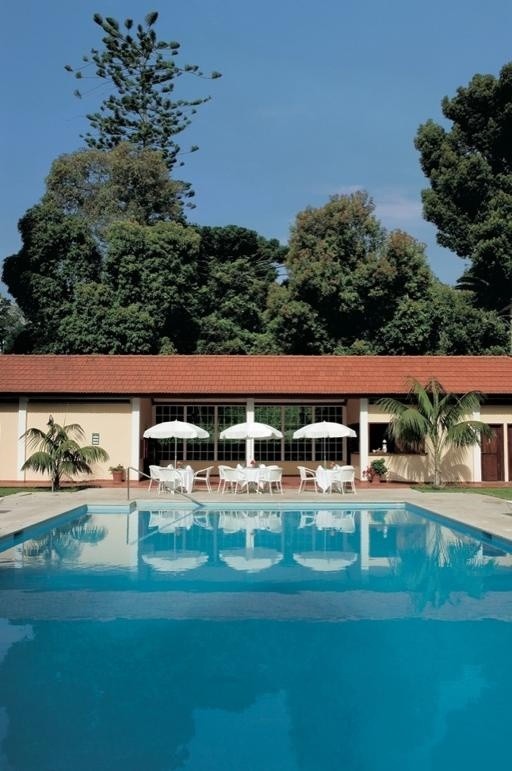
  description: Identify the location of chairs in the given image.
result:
[296,465,356,495]
[217,464,283,497]
[147,464,214,499]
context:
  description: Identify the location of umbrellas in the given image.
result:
[219,422,284,468]
[143,419,210,469]
[293,419,358,470]
[292,528,359,573]
[141,528,209,576]
[219,529,284,574]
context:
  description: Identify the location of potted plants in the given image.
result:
[366,458,387,483]
[108,464,125,483]
